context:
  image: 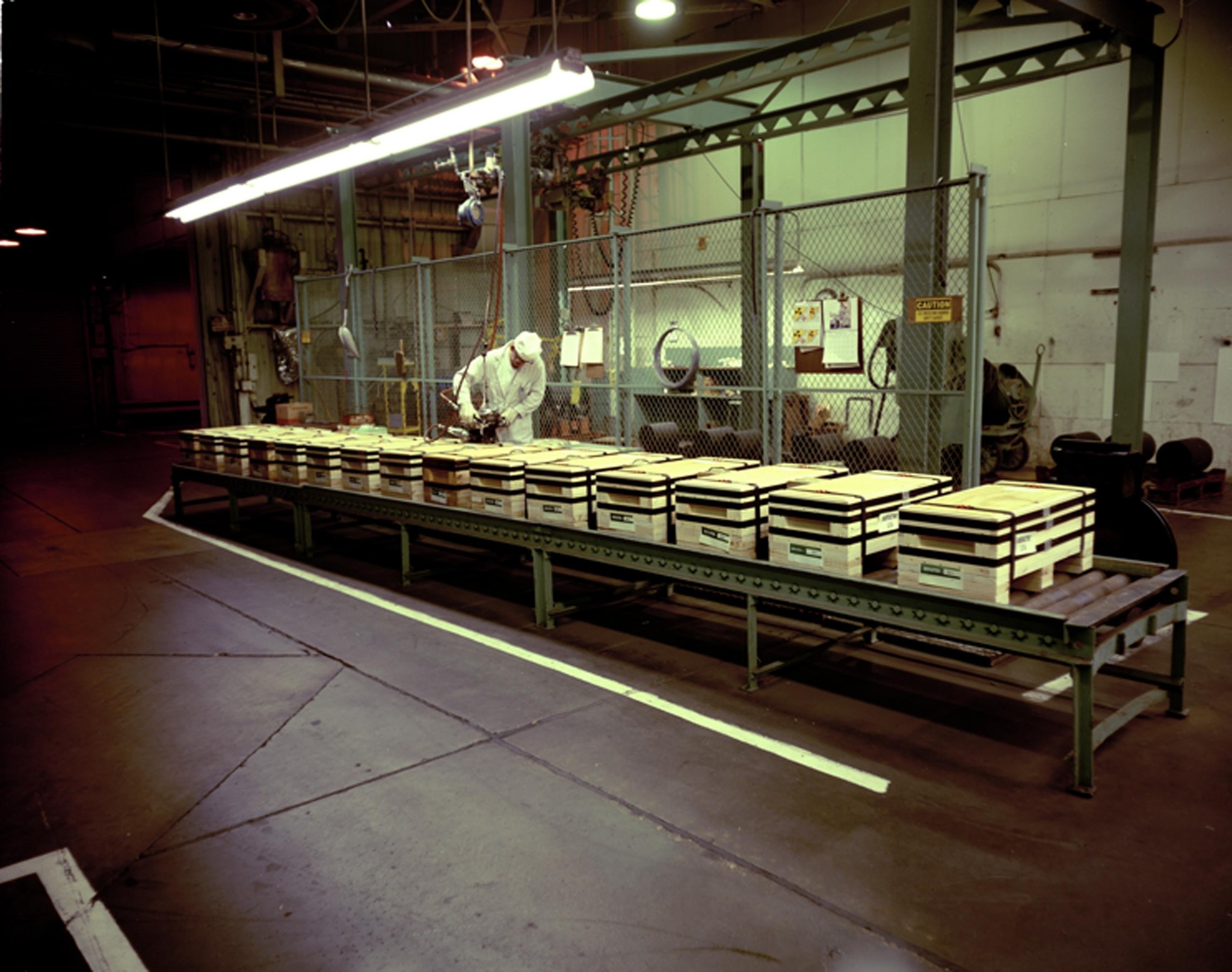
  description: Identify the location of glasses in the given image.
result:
[515,355,534,366]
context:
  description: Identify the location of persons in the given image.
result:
[449,329,547,445]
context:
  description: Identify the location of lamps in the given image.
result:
[160,3,598,225]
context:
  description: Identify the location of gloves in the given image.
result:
[500,408,519,427]
[459,403,481,429]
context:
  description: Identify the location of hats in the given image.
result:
[514,331,543,361]
